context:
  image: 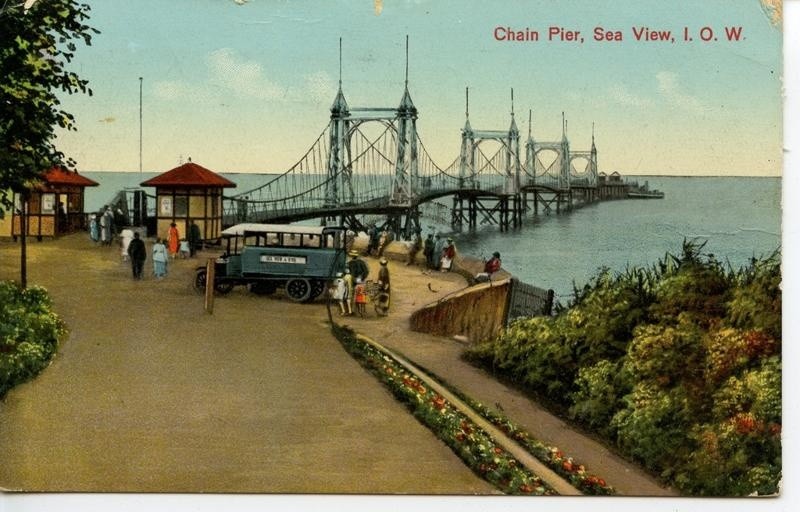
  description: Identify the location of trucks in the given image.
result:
[193,221,354,305]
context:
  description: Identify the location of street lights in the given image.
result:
[138,76,143,174]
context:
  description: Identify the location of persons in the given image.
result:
[330,250,391,317]
[89,205,200,279]
[474,252,502,279]
[58,202,66,230]
[364,225,455,272]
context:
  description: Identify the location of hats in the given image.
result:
[349,249,358,256]
